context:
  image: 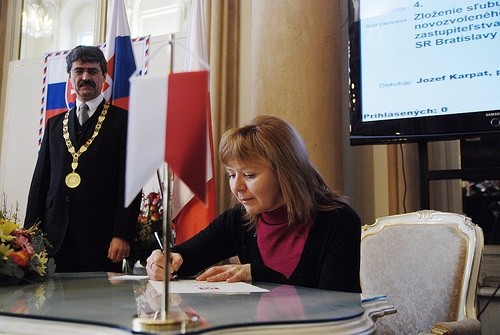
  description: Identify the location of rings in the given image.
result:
[150,262,155,269]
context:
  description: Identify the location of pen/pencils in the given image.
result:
[154,231,179,281]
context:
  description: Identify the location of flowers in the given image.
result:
[124,189,167,276]
[0,210,53,288]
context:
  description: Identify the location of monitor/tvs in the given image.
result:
[347,0,500,145]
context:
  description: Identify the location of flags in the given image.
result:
[100,0,137,112]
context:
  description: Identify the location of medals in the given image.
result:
[65,173,81,188]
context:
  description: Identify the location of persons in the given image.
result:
[23,45,143,274]
[145,114,363,294]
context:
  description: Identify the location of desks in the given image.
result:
[0,271,398,335]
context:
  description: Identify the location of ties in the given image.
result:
[78,103,89,126]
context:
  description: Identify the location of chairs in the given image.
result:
[359,209,484,335]
